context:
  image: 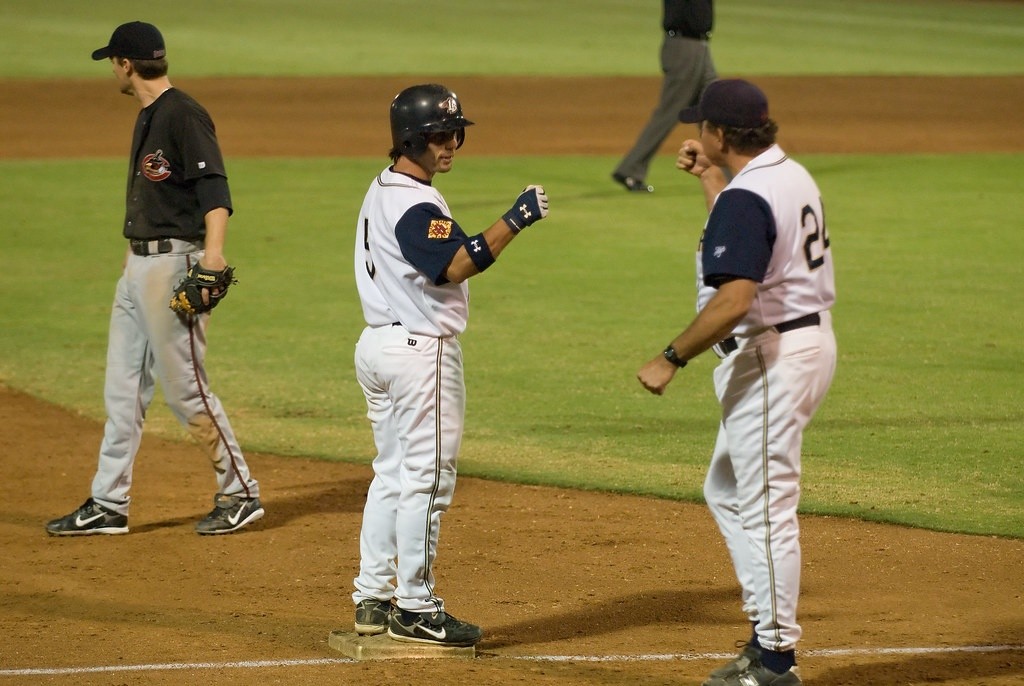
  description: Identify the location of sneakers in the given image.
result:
[46,497,131,534]
[195,497,265,533]
[701,664,802,686]
[388,604,481,646]
[709,639,762,679]
[355,596,402,634]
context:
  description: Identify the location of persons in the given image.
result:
[612,0,734,192]
[635,78,836,686]
[45,21,264,535]
[354,84,548,643]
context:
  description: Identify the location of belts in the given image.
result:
[129,238,172,255]
[392,321,402,326]
[719,313,820,355]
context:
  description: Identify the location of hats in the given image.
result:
[91,21,166,61]
[679,78,768,129]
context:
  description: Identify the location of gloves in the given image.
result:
[500,184,549,234]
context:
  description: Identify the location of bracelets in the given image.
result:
[663,345,687,369]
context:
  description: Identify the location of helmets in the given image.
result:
[390,84,475,153]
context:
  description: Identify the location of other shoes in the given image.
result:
[614,174,651,191]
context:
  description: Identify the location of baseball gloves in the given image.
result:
[168,260,233,323]
